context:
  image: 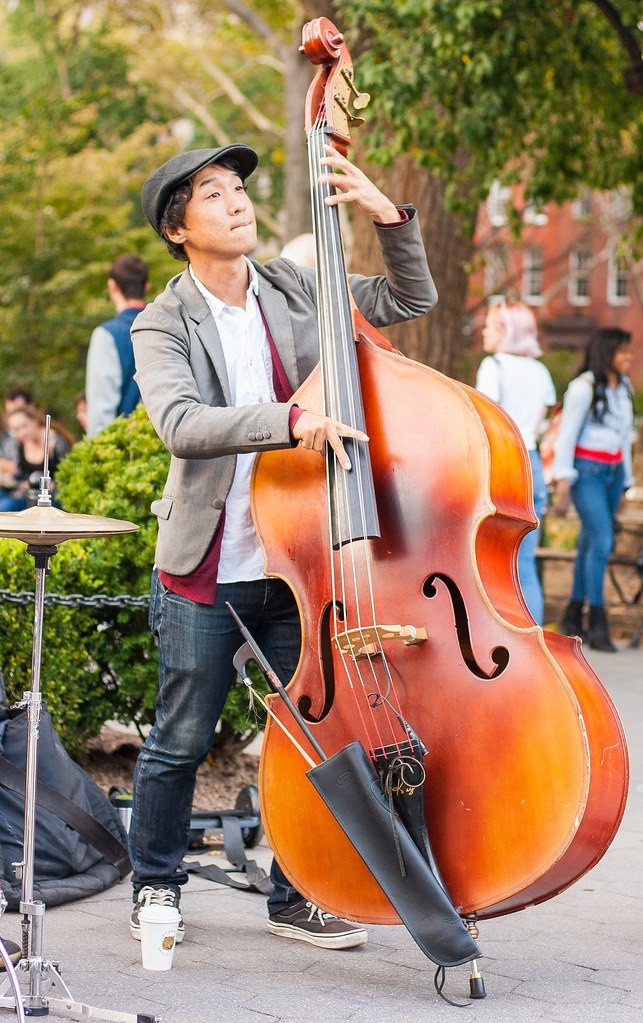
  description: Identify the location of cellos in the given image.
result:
[246,9,638,1008]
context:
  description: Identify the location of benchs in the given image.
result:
[534,548,642,606]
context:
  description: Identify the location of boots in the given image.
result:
[589,606,616,652]
[561,600,587,641]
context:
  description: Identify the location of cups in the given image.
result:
[137,906,181,971]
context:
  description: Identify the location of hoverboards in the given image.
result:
[100,785,265,849]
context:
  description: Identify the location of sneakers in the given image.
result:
[0,937,22,971]
[269,899,368,948]
[130,883,186,942]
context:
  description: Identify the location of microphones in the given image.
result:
[397,715,429,756]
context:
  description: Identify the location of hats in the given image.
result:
[141,143,258,235]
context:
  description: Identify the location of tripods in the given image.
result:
[0,543,162,1023]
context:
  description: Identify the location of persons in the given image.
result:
[474,294,557,627]
[0,252,153,513]
[550,327,638,652]
[129,139,439,953]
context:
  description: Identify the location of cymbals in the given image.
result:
[0,495,142,546]
